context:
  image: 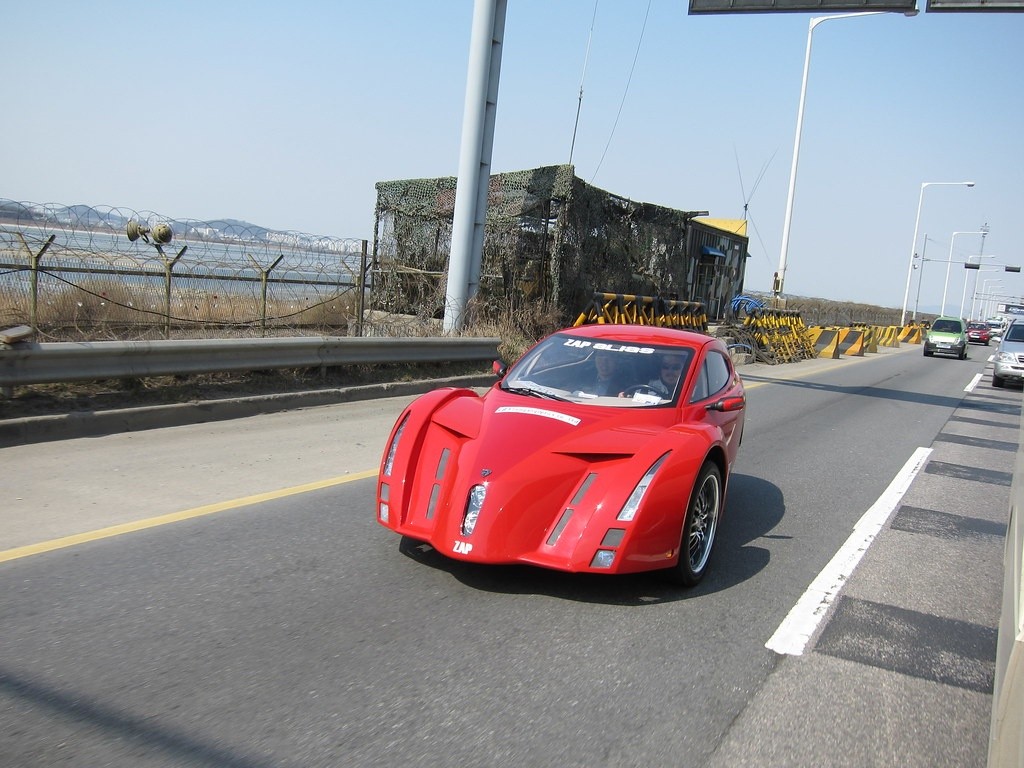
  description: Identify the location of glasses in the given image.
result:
[661,362,683,371]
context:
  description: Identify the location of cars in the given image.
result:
[992,318,1024,387]
[924,316,1008,360]
[376,324,746,584]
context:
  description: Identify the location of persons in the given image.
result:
[618,354,702,402]
[573,349,629,397]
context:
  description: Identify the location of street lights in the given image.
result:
[960,255,995,320]
[941,232,988,317]
[969,222,990,323]
[772,3,920,299]
[971,270,1005,322]
[900,182,976,327]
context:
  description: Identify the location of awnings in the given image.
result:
[702,246,725,257]
